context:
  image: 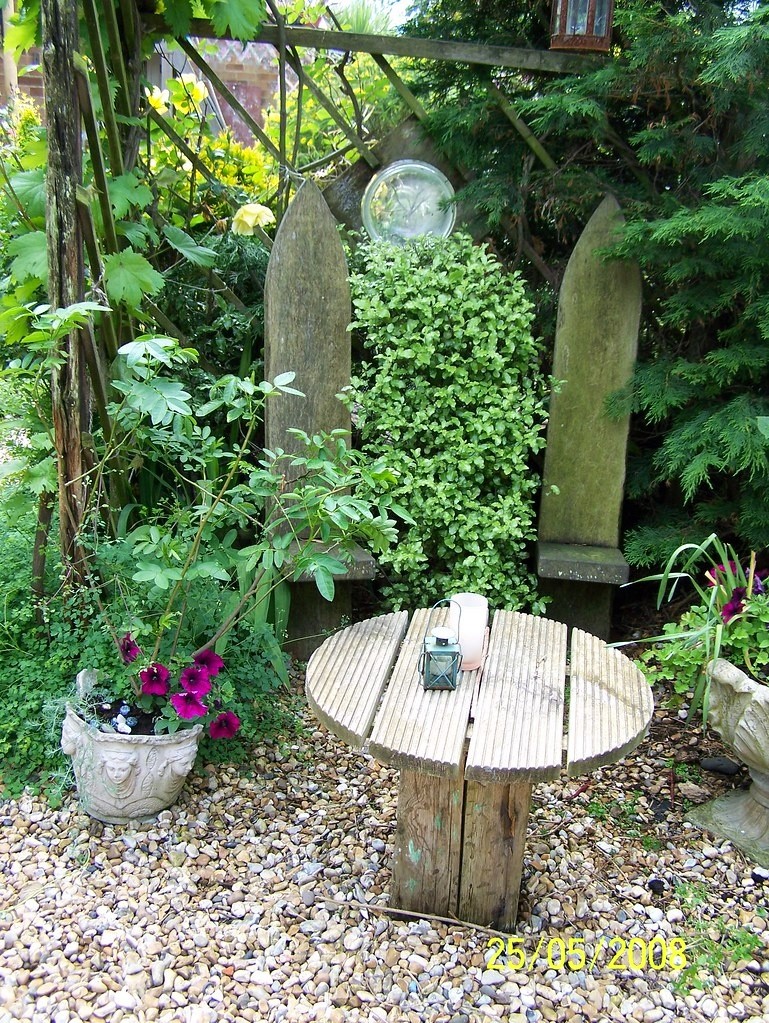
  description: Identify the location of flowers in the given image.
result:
[602,532,769,738]
[0,285,401,741]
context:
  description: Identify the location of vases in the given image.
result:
[60,668,206,825]
[681,657,769,869]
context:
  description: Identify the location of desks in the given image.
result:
[305,607,655,935]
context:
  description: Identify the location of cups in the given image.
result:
[449,592,488,671]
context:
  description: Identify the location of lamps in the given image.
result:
[548,0,615,55]
